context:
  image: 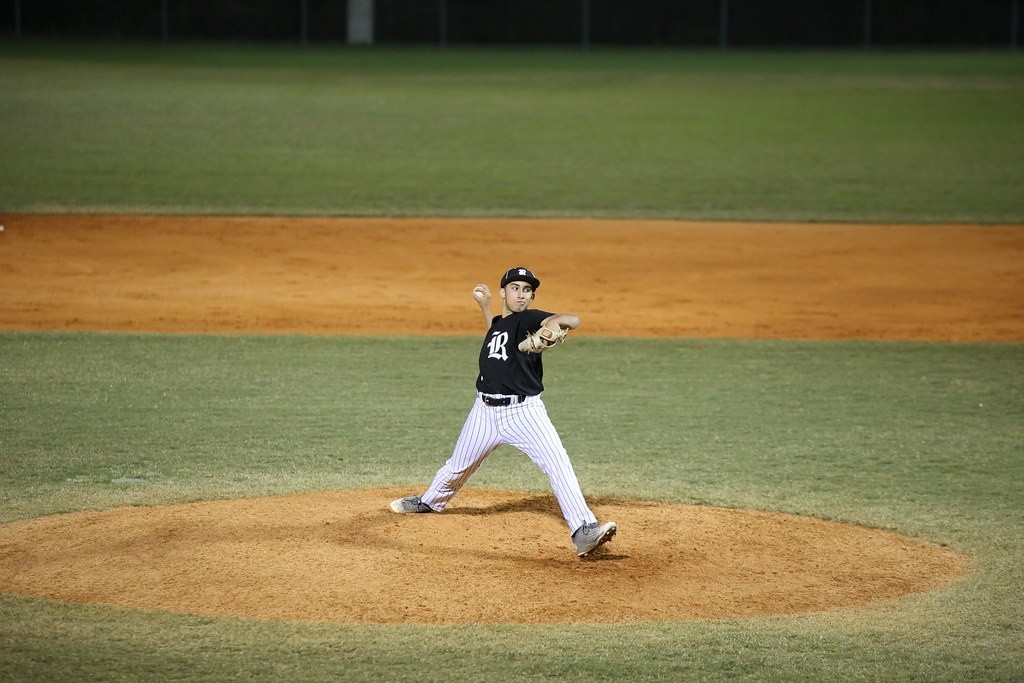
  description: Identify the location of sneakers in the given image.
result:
[574,522,616,557]
[391,495,434,513]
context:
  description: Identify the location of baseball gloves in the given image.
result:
[517,326,569,355]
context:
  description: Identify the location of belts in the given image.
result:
[482,393,526,406]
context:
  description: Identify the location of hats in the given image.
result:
[500,267,540,291]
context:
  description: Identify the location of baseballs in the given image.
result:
[473,286,484,300]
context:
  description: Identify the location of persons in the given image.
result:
[388,267,618,557]
